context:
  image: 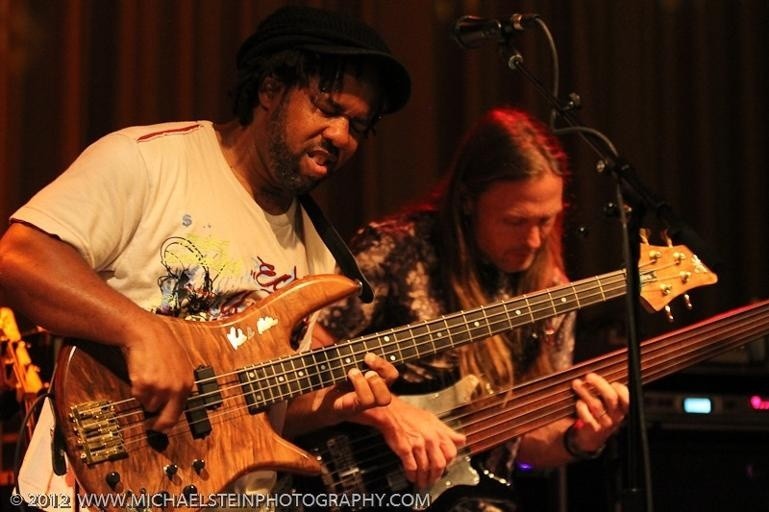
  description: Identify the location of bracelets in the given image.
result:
[562,421,608,464]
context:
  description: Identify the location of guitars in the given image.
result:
[47,219,718,510]
[277,298,768,512]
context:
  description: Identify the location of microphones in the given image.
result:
[443,14,543,51]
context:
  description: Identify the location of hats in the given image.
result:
[235,5,412,117]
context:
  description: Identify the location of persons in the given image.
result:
[281,105,636,511]
[2,1,402,512]
